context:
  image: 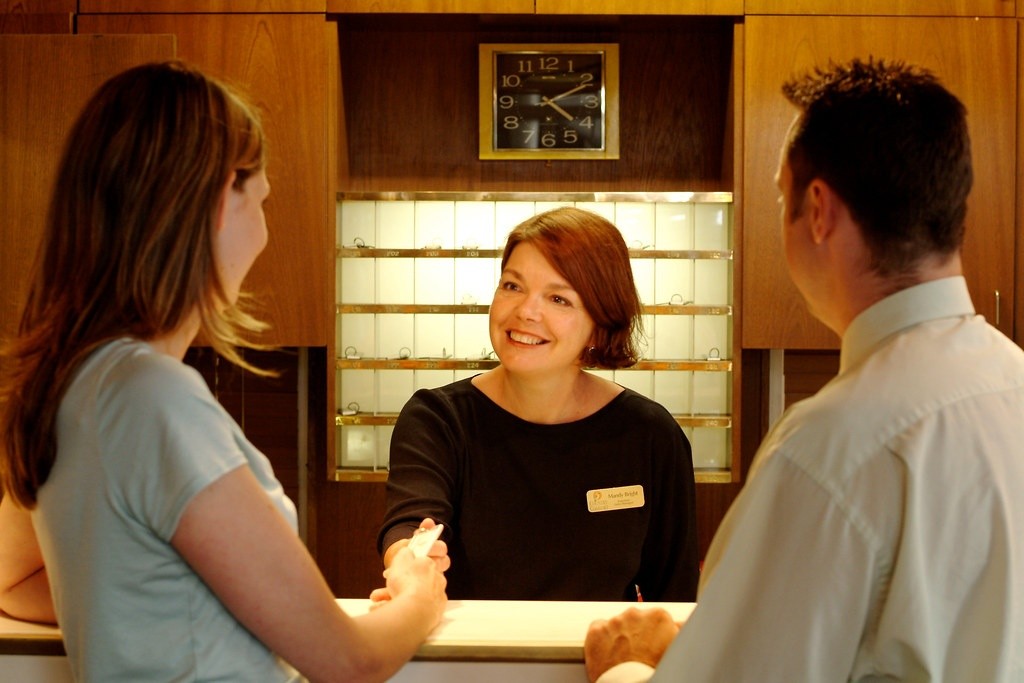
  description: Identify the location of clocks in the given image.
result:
[478,39,624,162]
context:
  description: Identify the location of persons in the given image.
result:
[583,54,1024,683]
[368,207,700,611]
[0,60,449,683]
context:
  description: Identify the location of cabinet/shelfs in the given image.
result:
[0,0,1024,349]
